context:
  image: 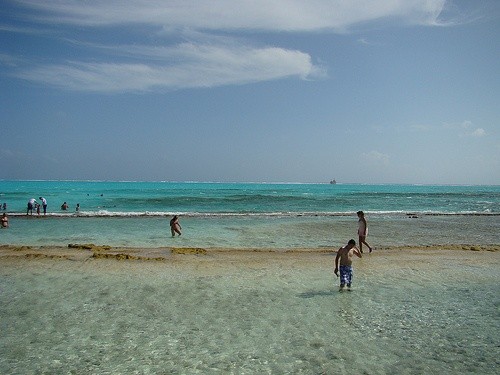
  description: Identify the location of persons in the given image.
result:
[76,204,80,211]
[27,197,40,217]
[357,211,373,253]
[3,202,6,209]
[39,197,47,216]
[334,239,362,288]
[170,215,182,236]
[61,202,67,210]
[0,212,8,228]
